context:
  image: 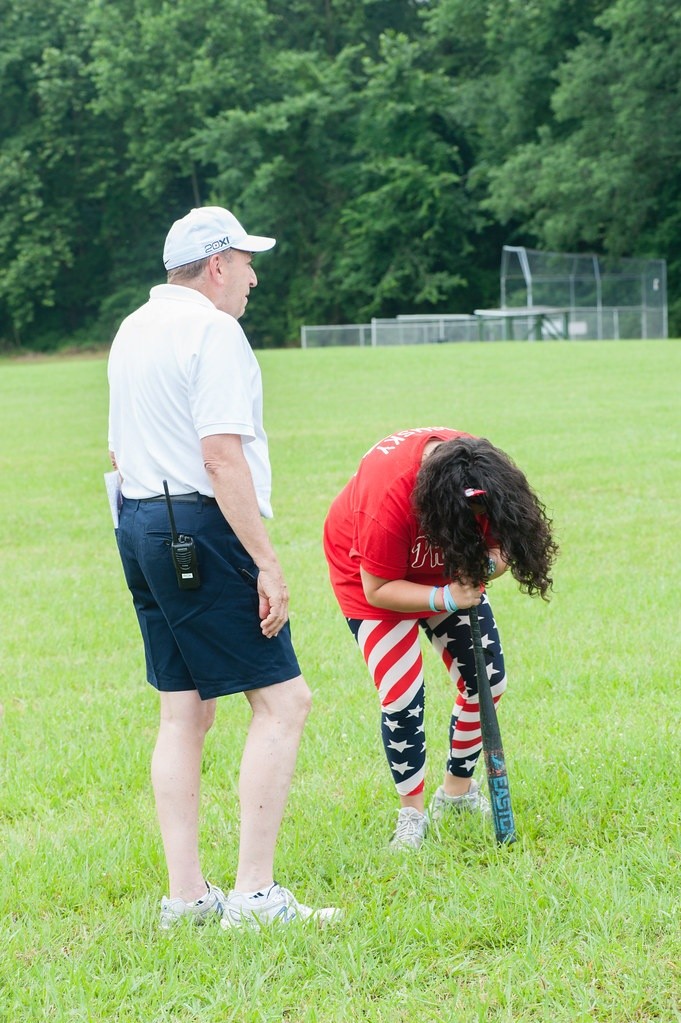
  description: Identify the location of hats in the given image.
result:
[163,207,277,271]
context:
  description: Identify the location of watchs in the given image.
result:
[486,556,496,577]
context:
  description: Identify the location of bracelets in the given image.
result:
[429,584,460,615]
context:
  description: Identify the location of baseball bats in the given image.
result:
[467,606,518,848]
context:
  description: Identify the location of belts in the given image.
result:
[140,491,219,505]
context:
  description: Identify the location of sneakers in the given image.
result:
[220,880,345,937]
[158,879,226,932]
[388,807,431,854]
[428,778,494,821]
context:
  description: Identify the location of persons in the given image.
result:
[106,204,348,933]
[322,426,560,854]
